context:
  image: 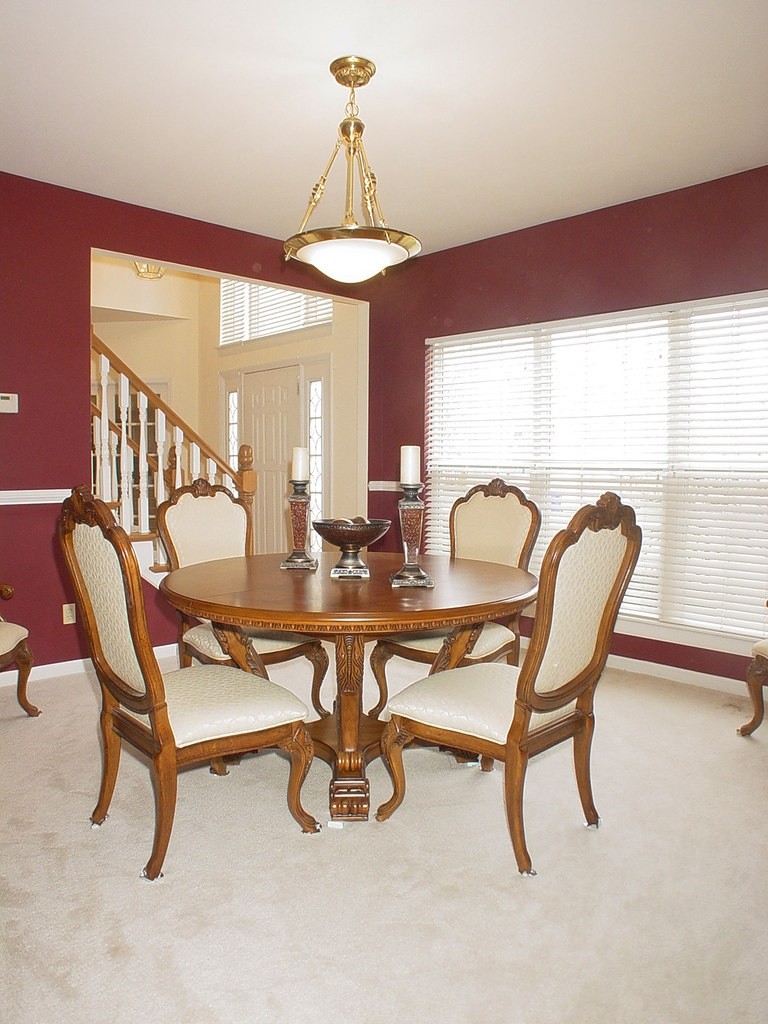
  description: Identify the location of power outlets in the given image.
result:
[62,604,75,624]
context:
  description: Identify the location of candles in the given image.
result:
[292,447,309,480]
[400,445,420,484]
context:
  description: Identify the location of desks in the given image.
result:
[158,553,540,826]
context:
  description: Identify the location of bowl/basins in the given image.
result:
[311,519,391,569]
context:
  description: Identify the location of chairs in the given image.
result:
[157,478,331,719]
[375,490,642,875]
[0,585,41,717]
[54,484,321,879]
[738,600,768,738]
[367,478,541,718]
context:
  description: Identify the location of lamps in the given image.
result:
[282,54,421,284]
[135,261,164,278]
